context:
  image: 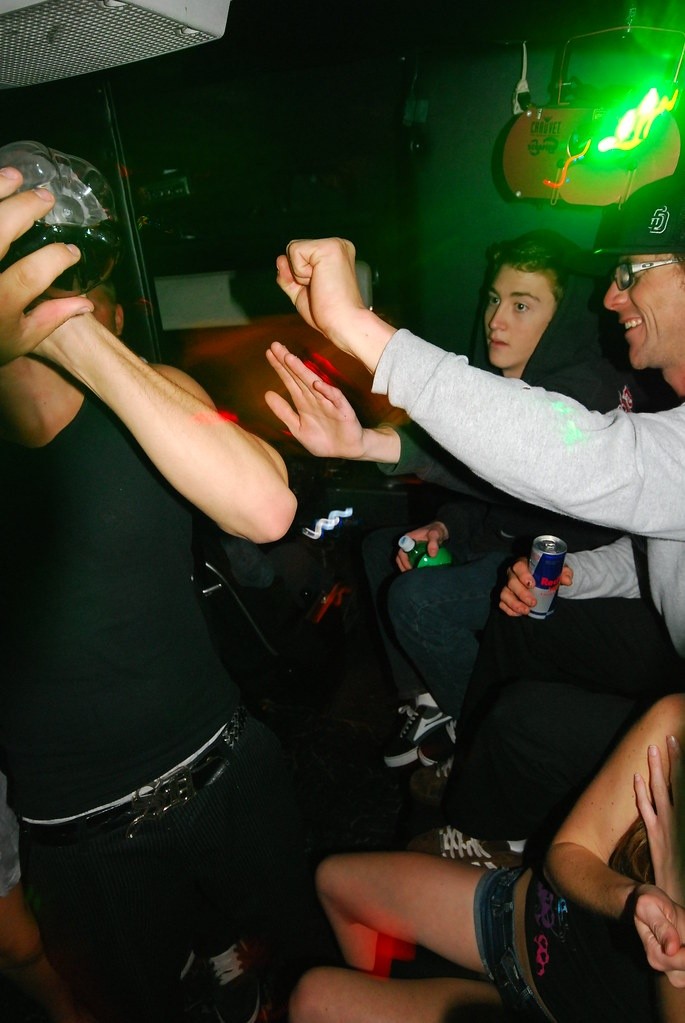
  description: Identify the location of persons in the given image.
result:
[0,140,685,1023]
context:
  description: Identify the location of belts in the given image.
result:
[14,700,250,847]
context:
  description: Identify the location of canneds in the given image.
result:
[526,535,567,620]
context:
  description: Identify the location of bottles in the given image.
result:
[398,535,452,569]
[0,139,120,315]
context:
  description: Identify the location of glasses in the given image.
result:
[612,255,685,291]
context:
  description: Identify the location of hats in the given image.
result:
[588,173,685,254]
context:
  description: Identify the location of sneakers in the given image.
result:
[406,825,519,870]
[384,688,452,767]
[408,752,455,806]
[417,716,459,767]
[194,924,260,1023]
